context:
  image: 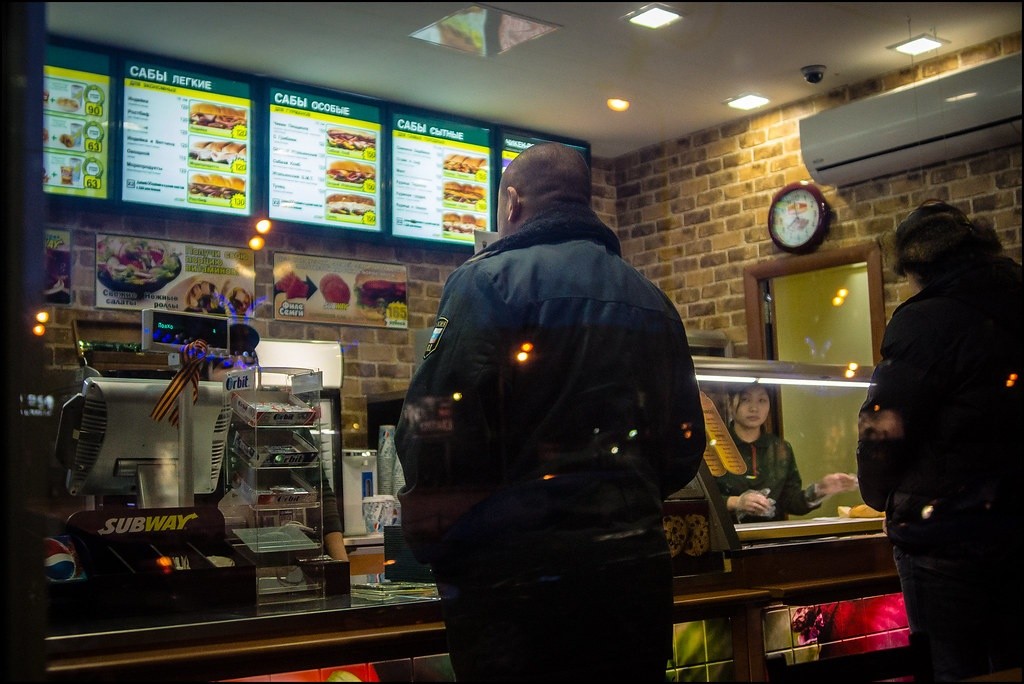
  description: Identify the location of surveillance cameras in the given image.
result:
[801,64,826,84]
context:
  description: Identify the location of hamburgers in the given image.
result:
[325,127,377,219]
[186,103,246,200]
[356,268,407,322]
[441,155,488,235]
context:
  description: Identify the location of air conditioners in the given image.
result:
[800,47,1022,188]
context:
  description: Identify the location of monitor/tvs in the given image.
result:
[55,376,233,497]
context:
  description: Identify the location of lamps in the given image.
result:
[605,95,632,112]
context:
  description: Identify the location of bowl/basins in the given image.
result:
[99,254,183,301]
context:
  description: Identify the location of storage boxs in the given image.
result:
[383,526,437,584]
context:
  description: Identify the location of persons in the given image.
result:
[855,203,1024,684]
[186,280,250,316]
[200,324,352,564]
[393,141,706,684]
[713,383,859,523]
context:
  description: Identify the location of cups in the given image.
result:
[361,425,407,536]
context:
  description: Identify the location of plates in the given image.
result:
[350,581,437,606]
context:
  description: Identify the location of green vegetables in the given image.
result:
[98,237,180,285]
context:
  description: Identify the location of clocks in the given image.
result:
[768,180,833,255]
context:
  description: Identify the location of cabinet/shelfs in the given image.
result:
[224,366,325,606]
